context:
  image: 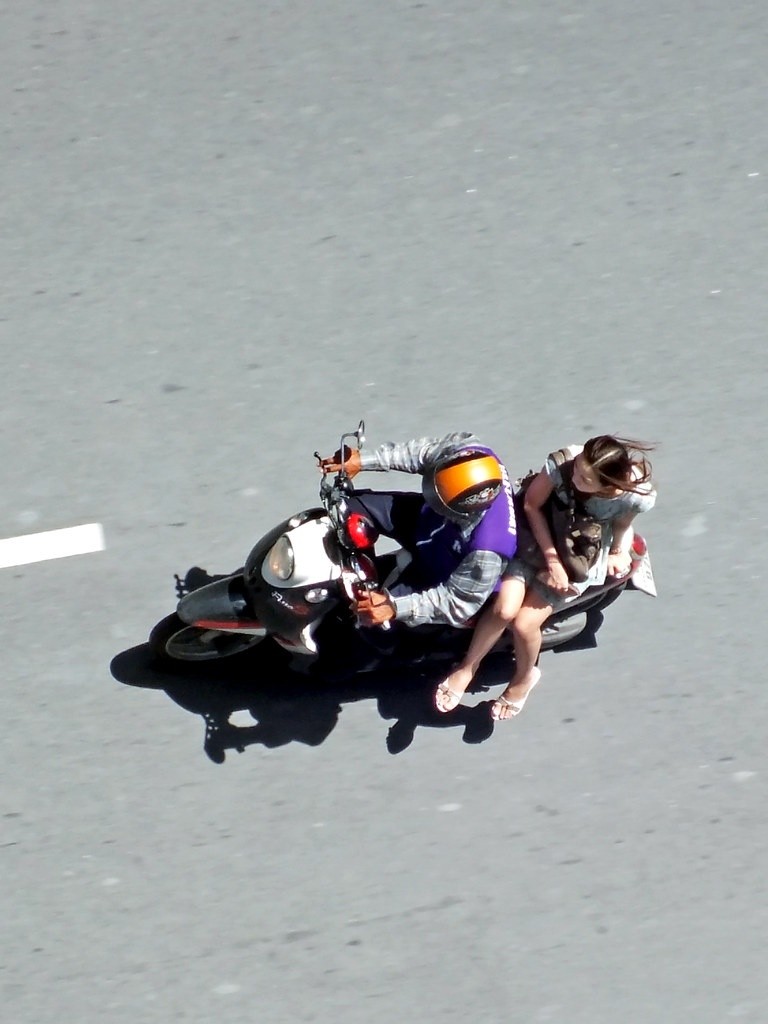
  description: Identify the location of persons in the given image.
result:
[435,435,658,721]
[317,432,517,626]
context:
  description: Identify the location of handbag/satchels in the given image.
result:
[514,448,602,582]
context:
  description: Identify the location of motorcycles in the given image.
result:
[147,421,657,696]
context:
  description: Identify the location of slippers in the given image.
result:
[491,666,541,721]
[436,669,461,713]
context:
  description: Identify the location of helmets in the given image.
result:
[423,443,503,521]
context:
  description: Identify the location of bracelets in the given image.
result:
[548,561,561,563]
